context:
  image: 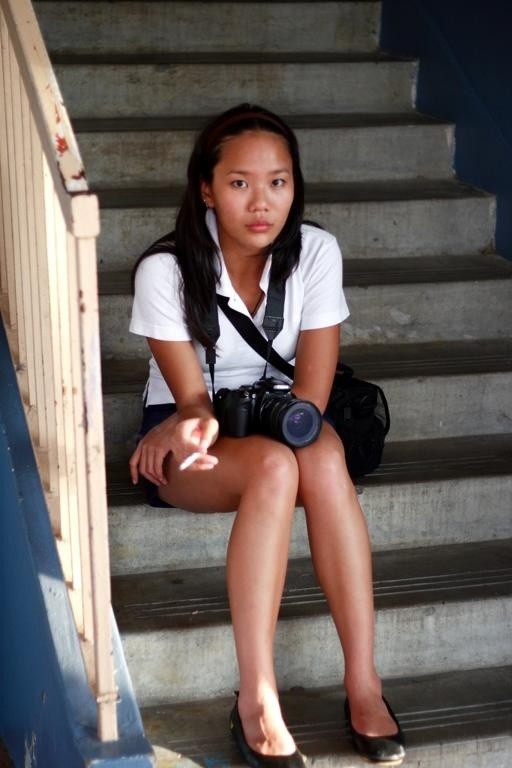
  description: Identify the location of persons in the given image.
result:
[121,98,414,768]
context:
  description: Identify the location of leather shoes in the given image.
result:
[228,686,308,768]
[342,691,409,767]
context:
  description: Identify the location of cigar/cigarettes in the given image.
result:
[178,450,209,471]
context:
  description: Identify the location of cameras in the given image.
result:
[213,377,323,448]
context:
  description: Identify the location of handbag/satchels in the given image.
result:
[322,358,392,485]
